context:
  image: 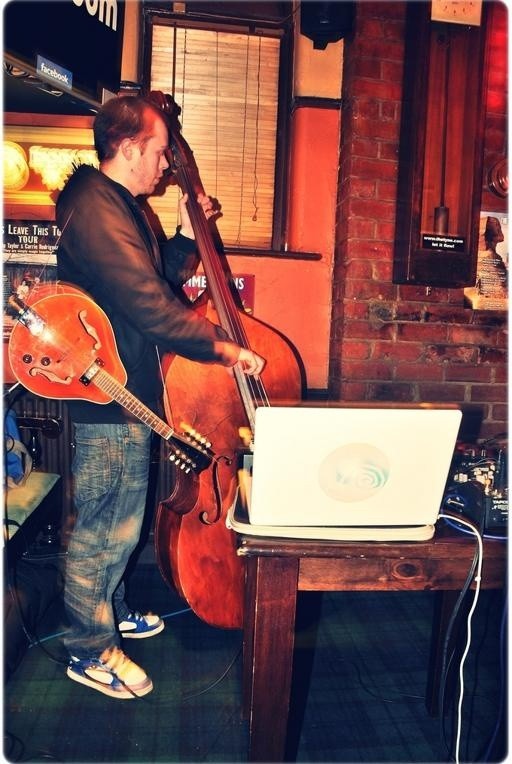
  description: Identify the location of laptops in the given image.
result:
[239,408,465,528]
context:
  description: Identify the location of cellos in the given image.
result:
[143,89,305,629]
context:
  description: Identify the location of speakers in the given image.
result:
[300,1,355,50]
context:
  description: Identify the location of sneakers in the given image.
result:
[66,648,154,700]
[120,613,165,640]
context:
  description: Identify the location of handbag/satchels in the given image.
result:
[5,408,33,491]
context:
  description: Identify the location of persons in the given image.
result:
[56,96,266,700]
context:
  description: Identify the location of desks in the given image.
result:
[228,456,507,764]
